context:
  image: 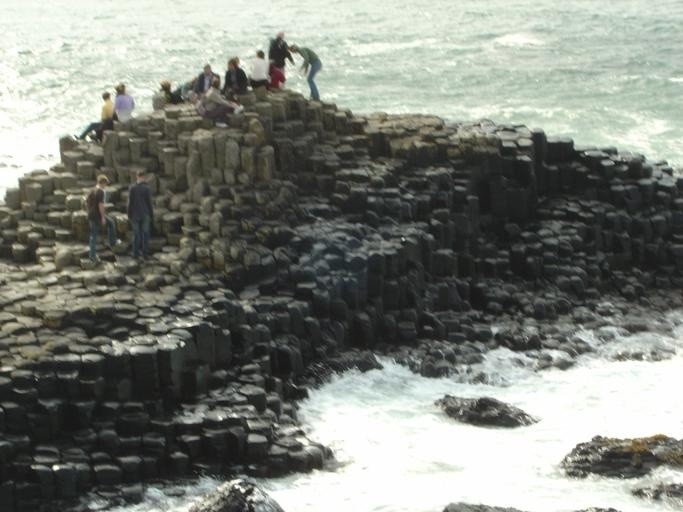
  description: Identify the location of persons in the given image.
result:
[127,171,157,260]
[74,30,294,141]
[85,174,122,262]
[288,44,323,102]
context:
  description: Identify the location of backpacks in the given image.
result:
[196,91,213,117]
